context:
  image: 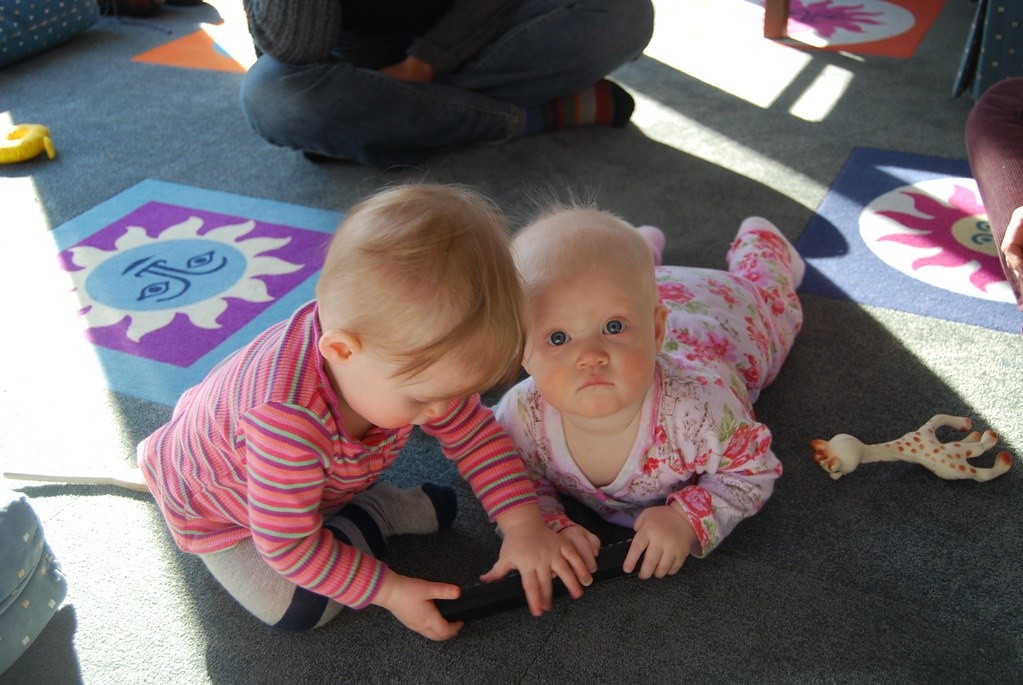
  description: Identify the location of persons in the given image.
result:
[493,209,805,579]
[136,185,593,642]
[240,0,655,170]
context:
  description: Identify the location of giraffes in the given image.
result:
[809,414,1011,484]
